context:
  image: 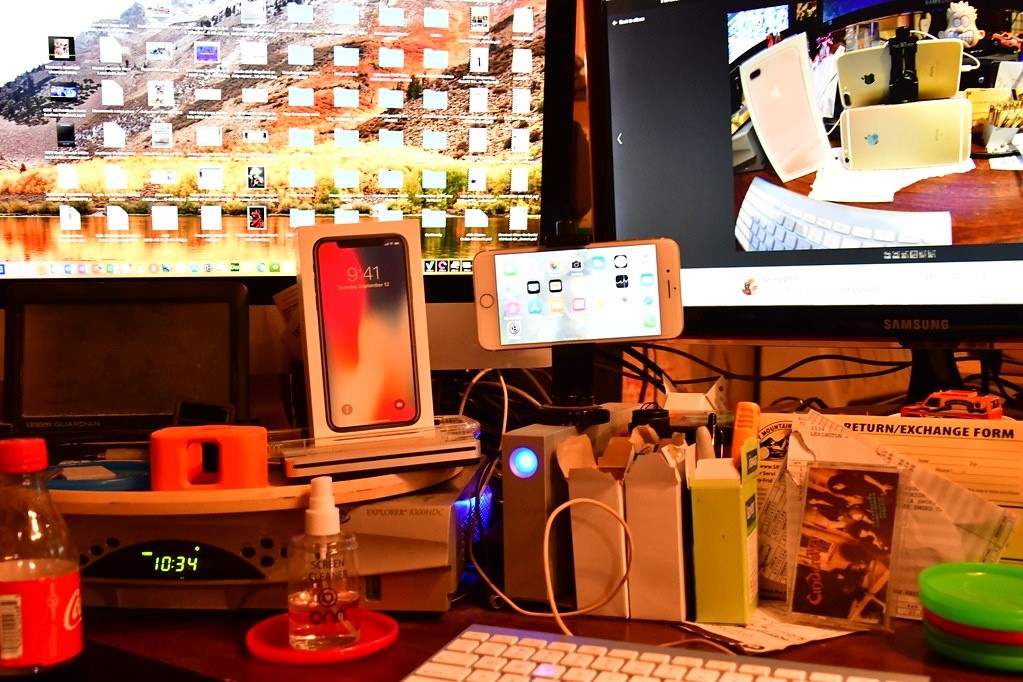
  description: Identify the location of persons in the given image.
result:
[938,2,986,47]
[806,473,894,601]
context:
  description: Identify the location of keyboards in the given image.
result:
[733,178,952,252]
[399,623,931,682]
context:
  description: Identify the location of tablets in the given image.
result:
[5,282,249,437]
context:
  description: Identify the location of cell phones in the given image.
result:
[836,39,974,170]
[312,231,422,433]
[474,238,685,349]
[747,44,826,178]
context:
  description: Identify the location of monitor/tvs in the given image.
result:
[725,2,797,76]
[816,0,981,36]
[581,0,1023,405]
[1,0,577,305]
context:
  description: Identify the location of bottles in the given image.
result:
[0,437,86,682]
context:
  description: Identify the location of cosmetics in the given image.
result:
[288,477,361,649]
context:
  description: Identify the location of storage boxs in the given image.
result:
[685,440,762,625]
[621,442,696,622]
[503,421,572,602]
[555,432,638,621]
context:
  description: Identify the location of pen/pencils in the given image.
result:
[696,412,728,460]
[989,95,1023,129]
